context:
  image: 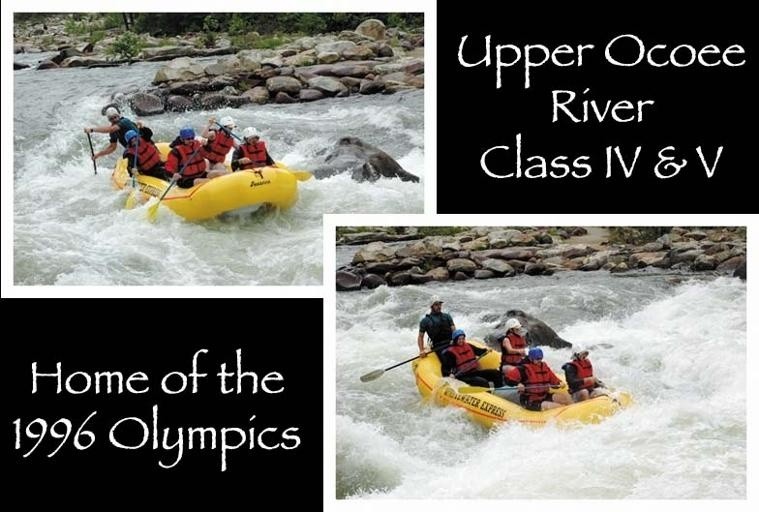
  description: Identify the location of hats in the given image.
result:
[220,116,237,129]
[428,295,444,306]
[507,319,523,330]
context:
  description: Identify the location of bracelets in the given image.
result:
[91,129,93,133]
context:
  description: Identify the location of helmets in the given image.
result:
[570,343,589,358]
[451,328,467,340]
[180,127,195,139]
[528,348,543,360]
[106,108,119,118]
[125,130,138,142]
[243,125,261,140]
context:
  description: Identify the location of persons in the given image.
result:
[231,127,277,172]
[499,317,531,387]
[504,347,573,412]
[440,329,504,394]
[165,127,217,189]
[561,348,596,400]
[201,116,239,170]
[418,295,457,364]
[84,106,141,161]
[122,121,173,182]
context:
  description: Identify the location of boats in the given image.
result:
[114,141,302,223]
[413,336,637,435]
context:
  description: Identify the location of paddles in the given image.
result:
[414,352,490,409]
[458,384,569,395]
[125,124,140,210]
[147,139,208,222]
[251,160,312,182]
[360,342,453,383]
